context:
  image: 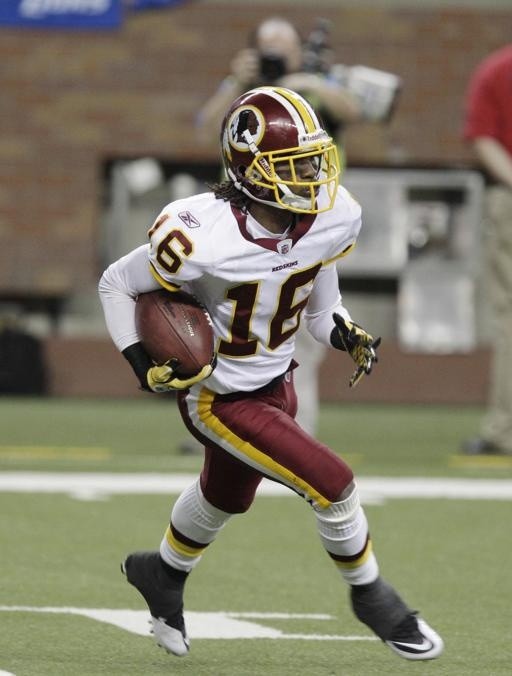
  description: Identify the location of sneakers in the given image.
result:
[121,551,190,657]
[351,577,445,661]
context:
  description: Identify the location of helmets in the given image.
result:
[221,86,340,214]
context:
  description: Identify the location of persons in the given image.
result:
[97,86,445,661]
[461,44,512,455]
[196,20,362,165]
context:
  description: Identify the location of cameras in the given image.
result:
[259,53,286,82]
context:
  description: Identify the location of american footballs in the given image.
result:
[134,289,218,378]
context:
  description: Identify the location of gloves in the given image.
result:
[331,312,382,388]
[146,351,217,394]
